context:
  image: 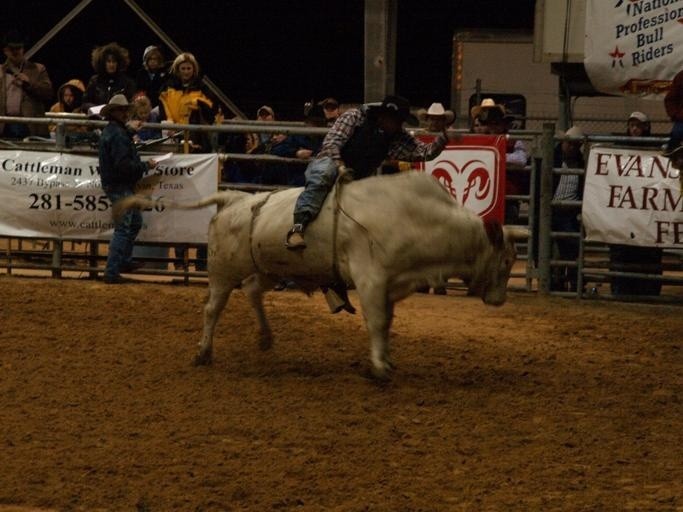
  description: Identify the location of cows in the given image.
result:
[109,166,534,385]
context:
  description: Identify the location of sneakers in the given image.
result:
[121,262,145,272]
[103,276,128,283]
[288,234,306,248]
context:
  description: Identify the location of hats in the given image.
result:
[417,103,454,127]
[471,99,505,121]
[368,96,419,127]
[99,95,134,116]
[258,105,273,115]
[630,111,649,123]
[557,126,587,143]
